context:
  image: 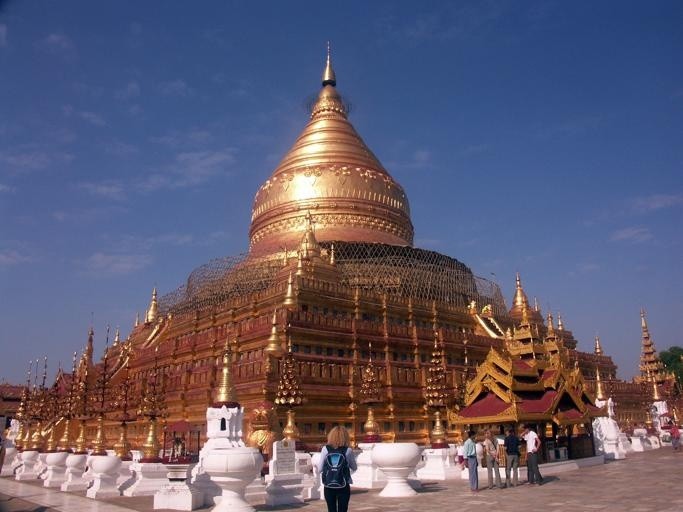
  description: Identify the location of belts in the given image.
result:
[467,456,477,458]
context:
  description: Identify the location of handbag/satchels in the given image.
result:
[517,450,521,457]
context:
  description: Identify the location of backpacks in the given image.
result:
[322,444,349,490]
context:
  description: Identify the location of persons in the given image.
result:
[667,419,681,453]
[519,423,544,486]
[316,425,357,512]
[462,430,481,496]
[483,429,503,490]
[501,427,521,489]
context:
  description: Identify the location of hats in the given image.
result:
[521,423,531,429]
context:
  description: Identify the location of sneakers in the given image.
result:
[526,480,543,486]
